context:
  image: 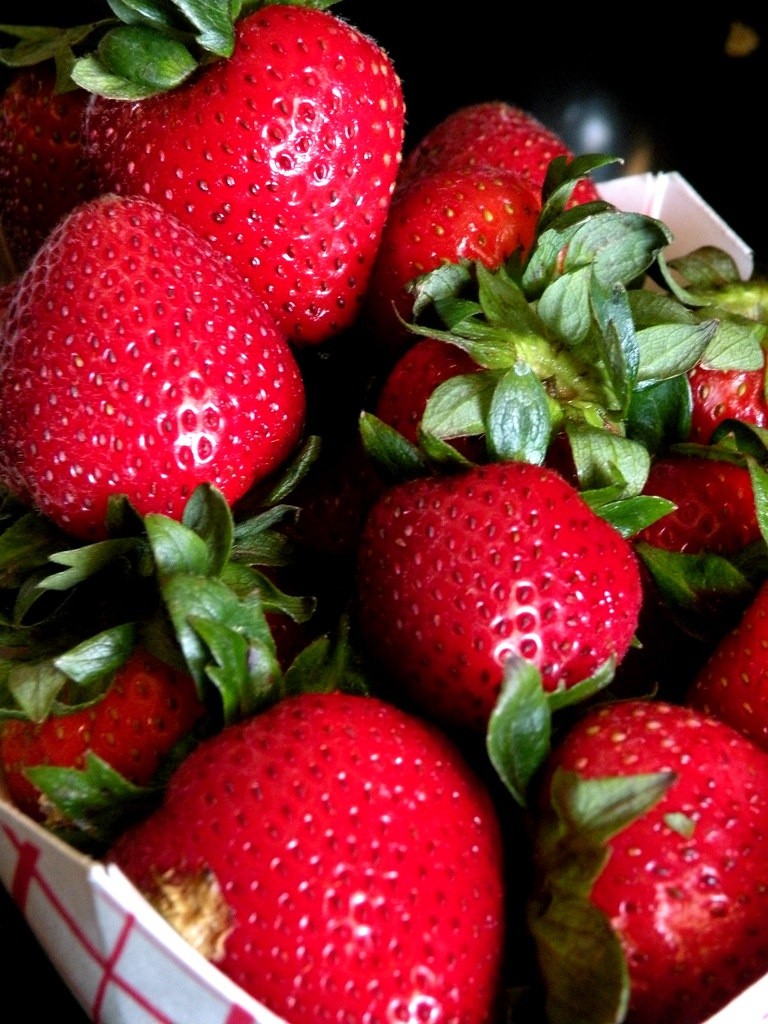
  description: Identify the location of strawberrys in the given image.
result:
[0,0,768,1024]
